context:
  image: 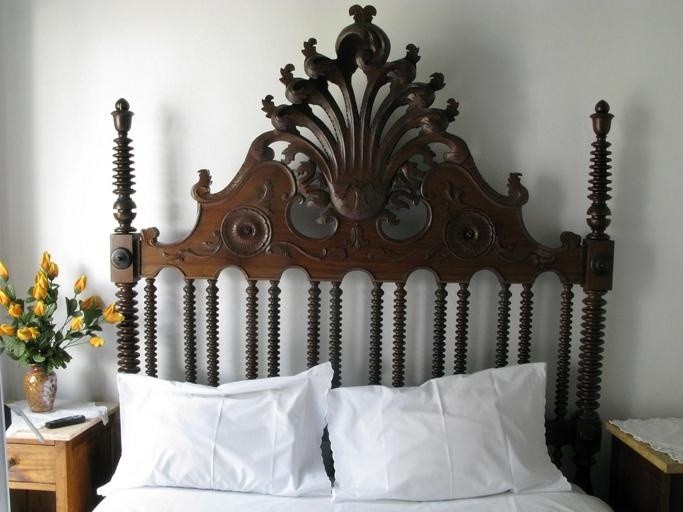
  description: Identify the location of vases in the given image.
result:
[23,366,57,413]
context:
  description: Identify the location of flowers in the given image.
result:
[0,251,126,375]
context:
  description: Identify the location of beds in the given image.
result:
[92,5,616,511]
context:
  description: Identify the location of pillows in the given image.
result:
[96,361,332,500]
[327,362,572,500]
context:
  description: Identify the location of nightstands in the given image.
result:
[3,398,120,512]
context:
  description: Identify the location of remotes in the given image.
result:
[44,414,86,429]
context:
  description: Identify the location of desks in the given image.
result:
[607,417,683,512]
